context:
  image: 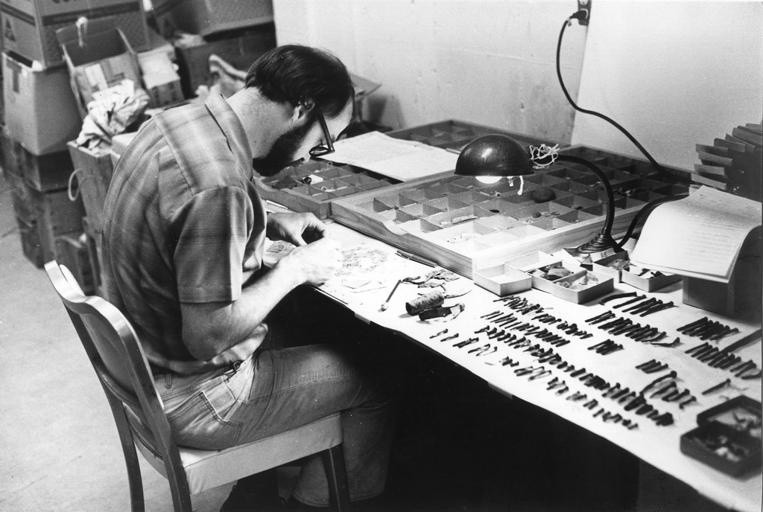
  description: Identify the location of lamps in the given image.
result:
[454,134,624,260]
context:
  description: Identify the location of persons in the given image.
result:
[97,42,389,512]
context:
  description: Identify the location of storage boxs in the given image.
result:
[178,35,277,95]
[150,0,274,40]
[2,26,176,156]
[0,0,151,70]
[11,187,86,268]
[3,133,77,193]
[66,139,112,221]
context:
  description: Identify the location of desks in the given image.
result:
[263,197,763,512]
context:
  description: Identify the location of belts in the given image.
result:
[147,361,245,376]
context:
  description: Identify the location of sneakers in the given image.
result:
[219,477,283,509]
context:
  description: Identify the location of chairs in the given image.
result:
[44,259,352,512]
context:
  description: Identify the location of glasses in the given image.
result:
[308,100,336,158]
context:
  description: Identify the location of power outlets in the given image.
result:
[578,1,592,26]
